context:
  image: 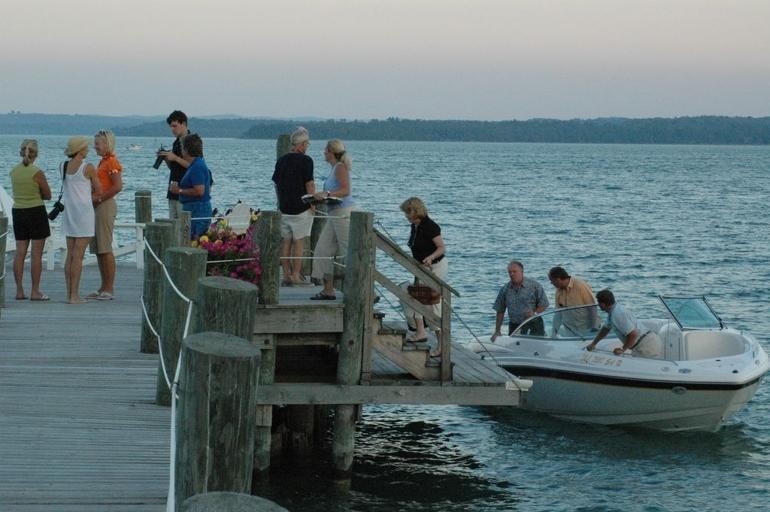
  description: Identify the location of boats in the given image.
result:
[460,293,770,442]
[123,143,143,151]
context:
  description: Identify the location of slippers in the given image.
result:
[309,290,339,303]
[87,287,115,300]
[12,292,51,303]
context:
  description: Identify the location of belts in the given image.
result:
[629,328,653,353]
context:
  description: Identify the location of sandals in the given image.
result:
[407,333,430,346]
[430,349,447,360]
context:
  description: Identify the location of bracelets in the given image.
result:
[431,255,436,261]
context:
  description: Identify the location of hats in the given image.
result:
[64,134,93,158]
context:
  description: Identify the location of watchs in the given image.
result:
[178,188,183,195]
[327,191,332,196]
[97,198,102,203]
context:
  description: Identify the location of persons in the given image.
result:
[587,289,664,360]
[169,135,213,240]
[491,261,548,342]
[160,111,192,219]
[272,126,316,287]
[310,140,380,303]
[549,267,601,336]
[87,128,123,299]
[10,138,52,300]
[59,135,102,304]
[400,197,448,357]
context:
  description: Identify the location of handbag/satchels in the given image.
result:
[406,261,444,307]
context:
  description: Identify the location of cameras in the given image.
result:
[153,147,171,169]
[48,201,64,220]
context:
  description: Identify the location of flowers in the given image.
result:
[189,199,263,288]
